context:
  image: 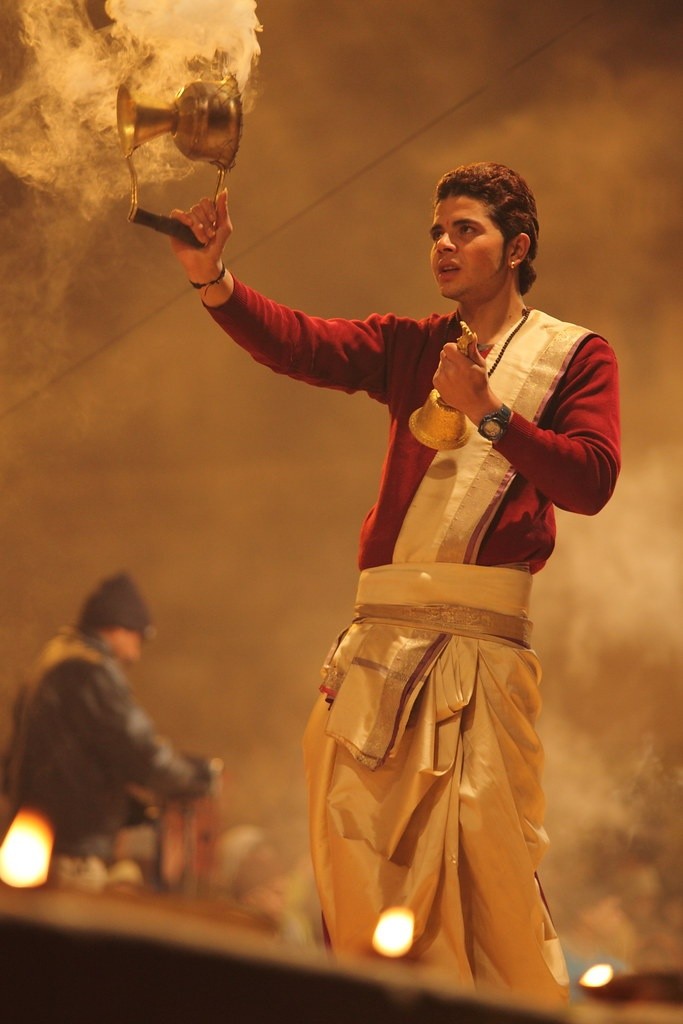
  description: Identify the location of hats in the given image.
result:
[81,573,151,634]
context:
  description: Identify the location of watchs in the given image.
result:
[478,403,512,443]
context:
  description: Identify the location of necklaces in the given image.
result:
[442,307,529,381]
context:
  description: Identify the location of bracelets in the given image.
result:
[189,262,225,297]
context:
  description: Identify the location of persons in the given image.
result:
[168,165,624,1005]
[2,572,219,894]
